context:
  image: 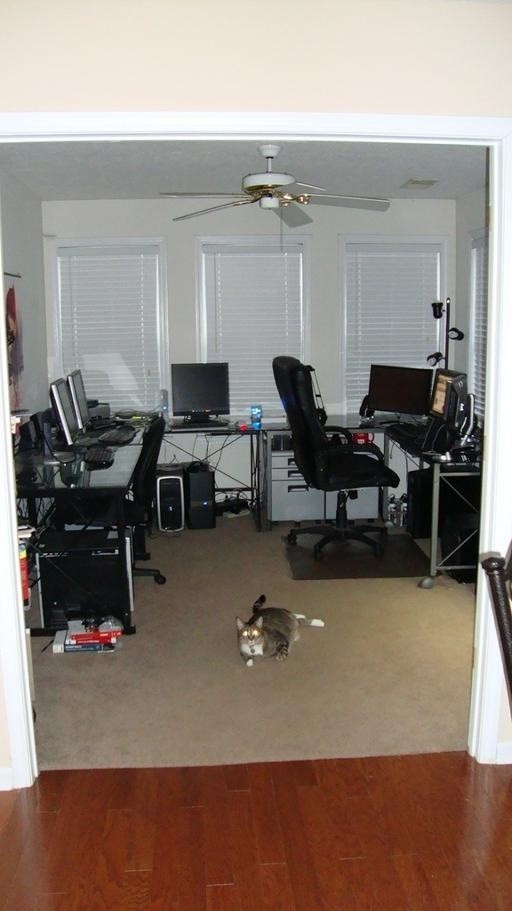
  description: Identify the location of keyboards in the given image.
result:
[84,445,115,468]
[98,429,137,446]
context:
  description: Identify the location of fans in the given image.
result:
[159,142,392,243]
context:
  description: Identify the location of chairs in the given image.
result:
[480,544,512,722]
[271,354,401,565]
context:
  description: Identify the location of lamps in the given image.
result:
[425,297,465,370]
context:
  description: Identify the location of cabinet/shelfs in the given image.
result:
[326,444,380,521]
[264,440,327,522]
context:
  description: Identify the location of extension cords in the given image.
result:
[223,507,250,518]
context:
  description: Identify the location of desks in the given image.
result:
[163,412,395,535]
[383,402,485,590]
[13,413,166,630]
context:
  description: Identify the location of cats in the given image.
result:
[236,594,325,666]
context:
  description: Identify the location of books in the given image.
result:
[52,617,124,653]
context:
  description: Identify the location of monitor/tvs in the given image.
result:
[66,370,91,431]
[41,379,79,465]
[441,374,476,450]
[367,364,430,424]
[429,368,466,420]
[171,363,230,428]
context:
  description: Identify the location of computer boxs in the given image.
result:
[184,460,215,529]
[35,529,133,629]
[155,462,184,533]
